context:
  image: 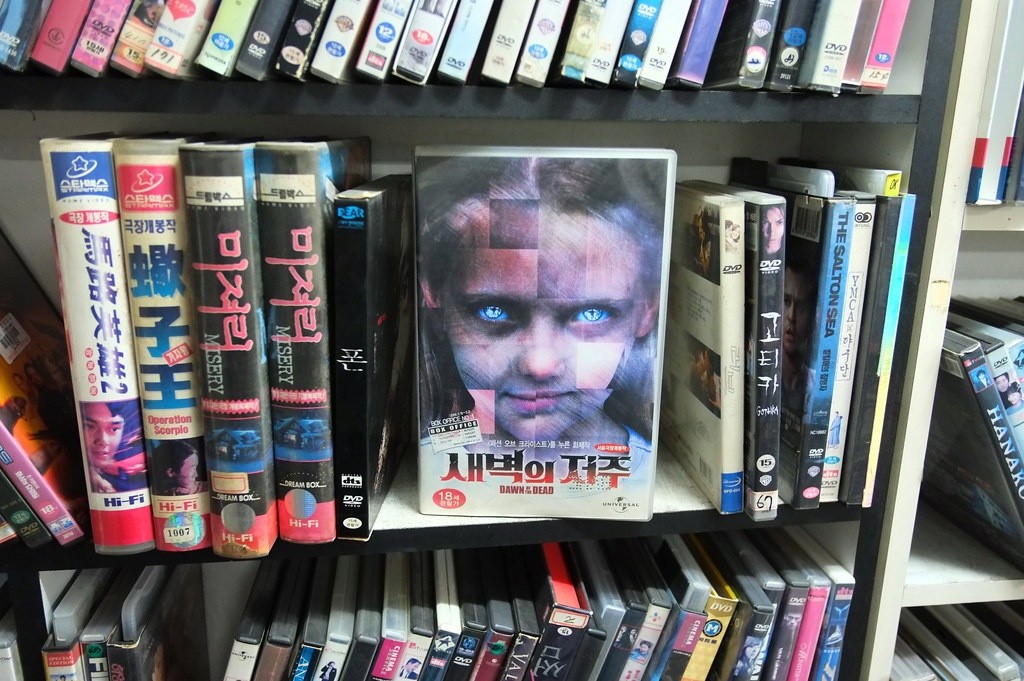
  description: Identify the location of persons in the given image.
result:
[80,401,144,493]
[781,610,802,656]
[695,347,717,402]
[415,157,668,505]
[320,660,337,681]
[399,658,422,680]
[154,440,208,497]
[614,625,652,663]
[975,368,1024,415]
[725,204,786,257]
[11,360,64,426]
[734,637,764,678]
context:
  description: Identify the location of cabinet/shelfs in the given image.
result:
[862,0,1024,681]
[0,0,962,681]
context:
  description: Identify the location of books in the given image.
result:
[0,515,857,681]
[889,599,1024,681]
[960,1,1024,209]
[935,290,1024,578]
[1,130,923,559]
[0,0,911,96]
